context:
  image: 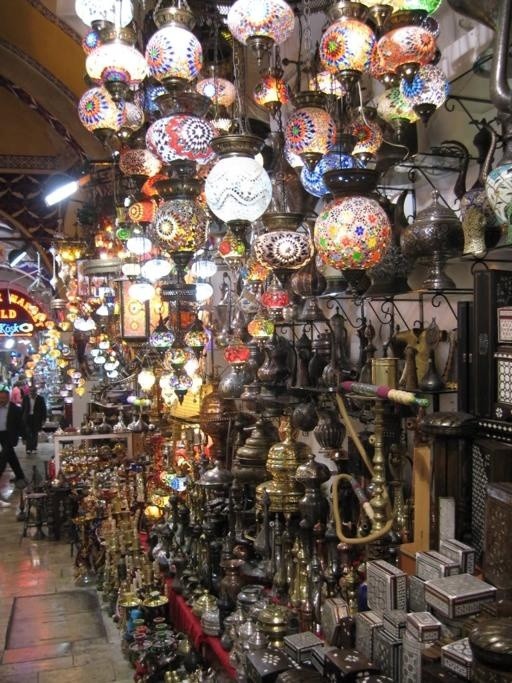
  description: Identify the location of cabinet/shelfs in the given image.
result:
[114,52,512,683]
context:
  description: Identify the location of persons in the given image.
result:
[0,371,46,483]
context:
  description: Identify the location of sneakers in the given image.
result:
[26,449,37,454]
[10,476,25,483]
[0,500,11,508]
